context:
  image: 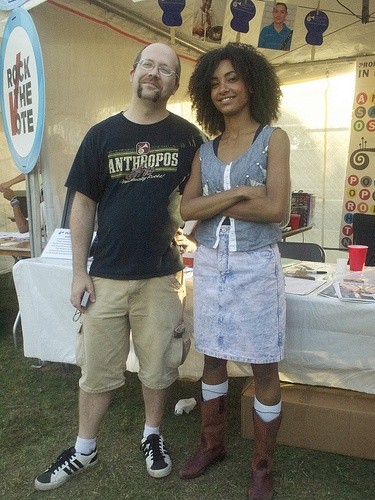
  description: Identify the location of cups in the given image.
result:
[289,214,301,229]
[183,254,194,268]
[348,245,368,272]
[336,258,349,271]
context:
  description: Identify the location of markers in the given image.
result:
[306,270,327,273]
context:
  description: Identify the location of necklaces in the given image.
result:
[3,186,46,259]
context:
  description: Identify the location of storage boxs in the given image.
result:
[240,375,375,458]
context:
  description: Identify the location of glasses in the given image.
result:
[135,60,180,80]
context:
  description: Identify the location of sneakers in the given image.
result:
[35,446,98,491]
[139,433,172,478]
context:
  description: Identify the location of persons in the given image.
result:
[179,42,292,500]
[258,2,293,51]
[35,43,212,491]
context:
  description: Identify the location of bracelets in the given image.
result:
[10,196,20,207]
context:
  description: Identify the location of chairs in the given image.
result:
[353,213,375,266]
[279,241,326,263]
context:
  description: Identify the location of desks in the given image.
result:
[281,225,312,242]
[12,253,375,396]
[0,233,32,288]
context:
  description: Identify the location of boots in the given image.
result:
[177,394,228,479]
[247,406,283,500]
